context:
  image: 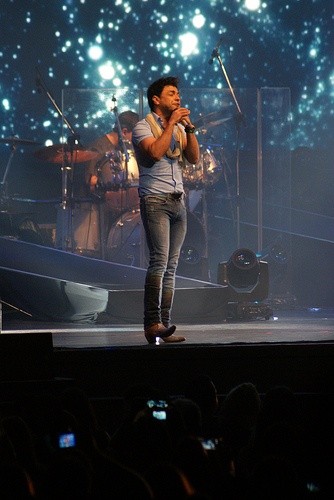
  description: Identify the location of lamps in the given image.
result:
[218,248,275,320]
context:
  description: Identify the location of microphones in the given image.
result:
[181,119,195,134]
[209,38,223,65]
[36,65,41,95]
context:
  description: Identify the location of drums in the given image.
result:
[54,195,101,259]
[183,145,223,190]
[94,148,140,188]
[106,210,151,270]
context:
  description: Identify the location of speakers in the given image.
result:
[0,266,109,320]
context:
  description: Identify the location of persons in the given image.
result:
[18,376,275,495]
[83,108,144,263]
[130,77,200,348]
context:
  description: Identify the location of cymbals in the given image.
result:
[1,137,40,146]
[35,143,100,164]
[194,118,232,132]
[192,111,217,123]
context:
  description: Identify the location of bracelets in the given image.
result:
[185,124,195,134]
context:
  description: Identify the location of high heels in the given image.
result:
[162,334,186,342]
[145,323,176,345]
